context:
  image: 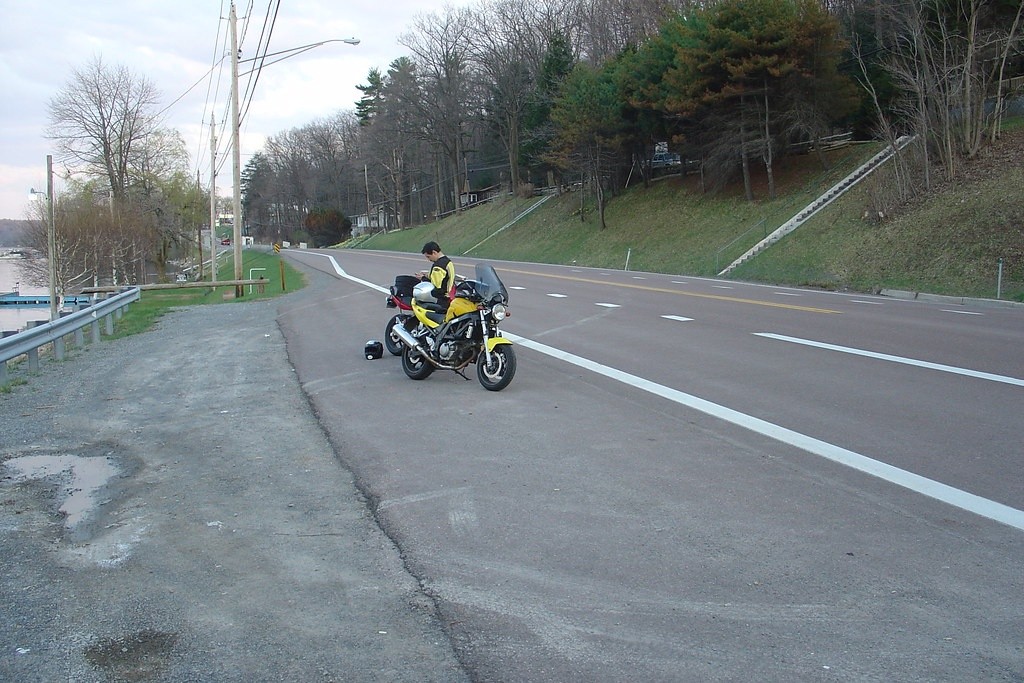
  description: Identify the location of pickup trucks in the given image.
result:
[641,152,683,165]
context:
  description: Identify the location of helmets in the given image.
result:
[412,281,439,303]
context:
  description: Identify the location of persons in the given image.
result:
[414,241,455,298]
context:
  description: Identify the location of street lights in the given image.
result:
[233,37,360,301]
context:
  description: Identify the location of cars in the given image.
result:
[221,239,230,245]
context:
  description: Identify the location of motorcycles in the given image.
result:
[385,264,518,391]
